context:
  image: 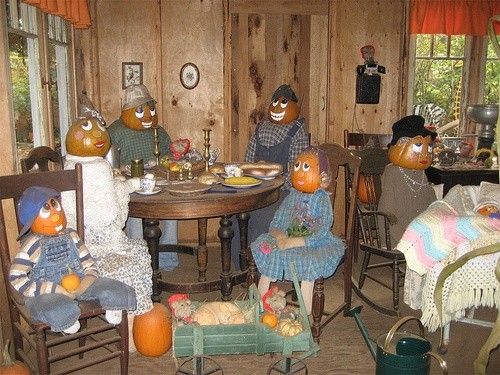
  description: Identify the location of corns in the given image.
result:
[224,177,260,184]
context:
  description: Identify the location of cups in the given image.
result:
[224,163,240,176]
[103,144,120,168]
[140,179,156,192]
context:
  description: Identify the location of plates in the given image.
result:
[165,182,210,196]
[211,171,285,189]
[126,170,169,195]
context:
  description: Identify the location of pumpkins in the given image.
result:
[192,302,245,324]
[62,267,80,290]
[280,318,303,336]
[358,174,383,202]
[134,303,172,357]
[262,312,278,328]
[0,340,34,375]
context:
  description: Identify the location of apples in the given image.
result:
[169,162,180,171]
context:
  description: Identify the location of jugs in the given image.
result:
[203,147,220,166]
[343,305,448,375]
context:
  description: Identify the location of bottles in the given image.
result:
[132,158,144,177]
[178,167,195,180]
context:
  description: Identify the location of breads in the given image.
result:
[240,162,283,176]
[198,165,226,184]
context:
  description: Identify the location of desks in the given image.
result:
[128,162,285,303]
[424,163,500,199]
[396,183,500,355]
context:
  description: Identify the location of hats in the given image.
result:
[15,185,60,241]
[78,89,108,124]
[272,83,298,102]
[386,115,437,146]
[121,81,158,111]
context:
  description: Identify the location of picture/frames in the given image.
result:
[122,61,143,89]
[180,63,200,89]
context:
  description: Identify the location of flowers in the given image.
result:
[273,200,326,251]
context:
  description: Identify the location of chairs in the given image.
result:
[248,142,362,344]
[344,129,392,262]
[21,146,65,174]
[347,146,406,316]
[0,163,128,375]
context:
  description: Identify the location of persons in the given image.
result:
[378,115,438,250]
[9,186,136,333]
[250,147,345,317]
[229,84,310,271]
[105,84,179,272]
[61,111,153,354]
